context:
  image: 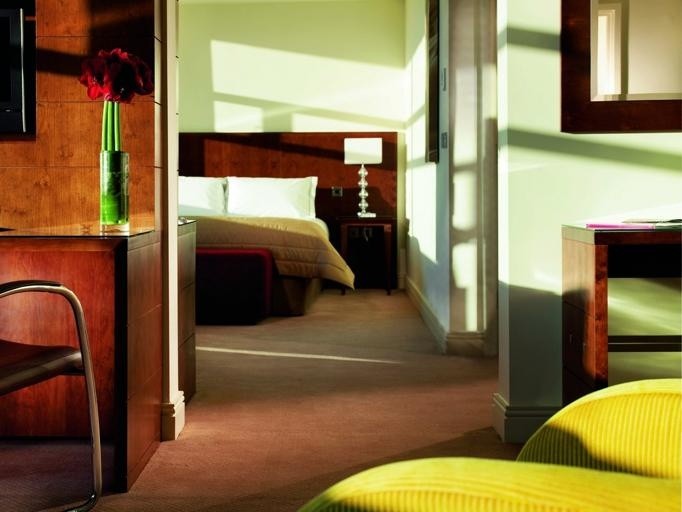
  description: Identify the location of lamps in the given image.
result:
[343,137,383,219]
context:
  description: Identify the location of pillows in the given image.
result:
[178,176,319,219]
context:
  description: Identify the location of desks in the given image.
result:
[561,221,681,407]
[336,217,395,296]
[1,225,163,492]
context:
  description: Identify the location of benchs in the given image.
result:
[195,247,273,326]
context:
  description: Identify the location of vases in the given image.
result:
[99,152,130,236]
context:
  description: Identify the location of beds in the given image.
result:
[177,214,330,317]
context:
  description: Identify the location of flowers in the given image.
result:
[75,49,154,222]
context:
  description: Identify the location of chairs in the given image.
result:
[1,280,103,512]
